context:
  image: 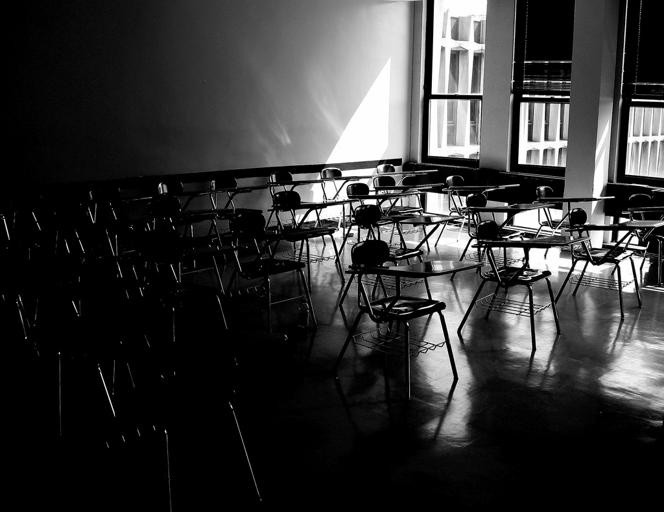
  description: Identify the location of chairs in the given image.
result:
[0,160,663,510]
